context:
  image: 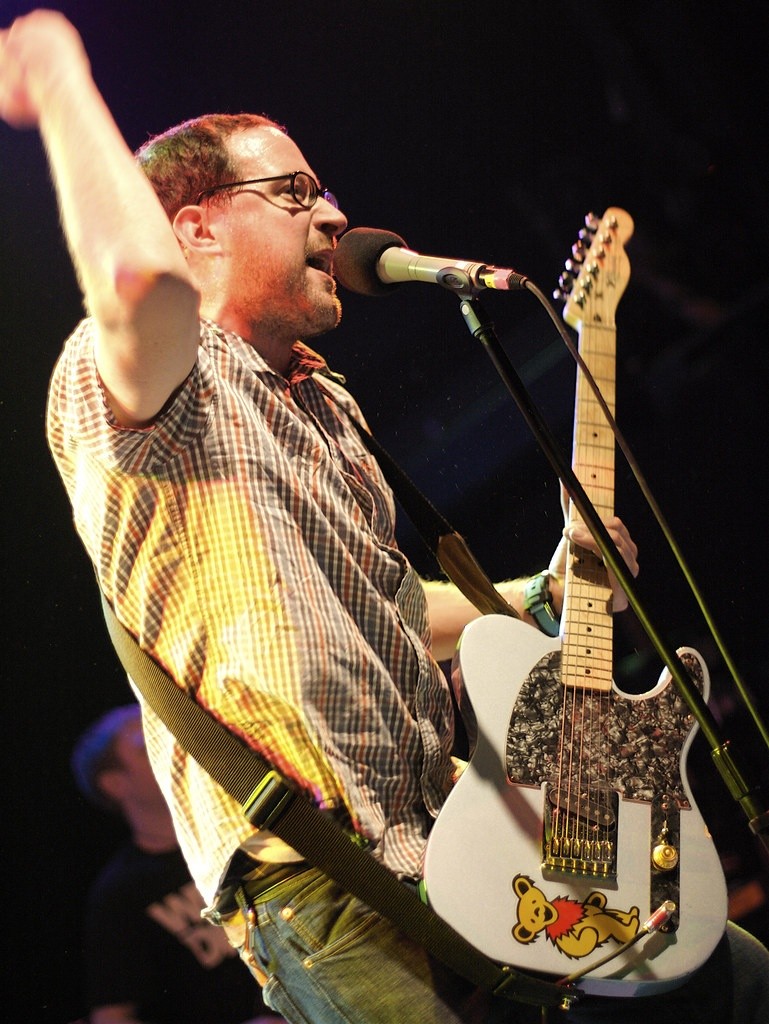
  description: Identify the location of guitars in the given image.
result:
[423,206,729,999]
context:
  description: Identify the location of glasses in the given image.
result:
[196,171,339,211]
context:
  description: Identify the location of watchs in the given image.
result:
[523,571,560,636]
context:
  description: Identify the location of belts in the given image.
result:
[220,858,315,917]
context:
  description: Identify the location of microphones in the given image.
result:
[331,225,530,292]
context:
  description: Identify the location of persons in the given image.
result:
[0,6,769,1024]
[75,705,296,1024]
